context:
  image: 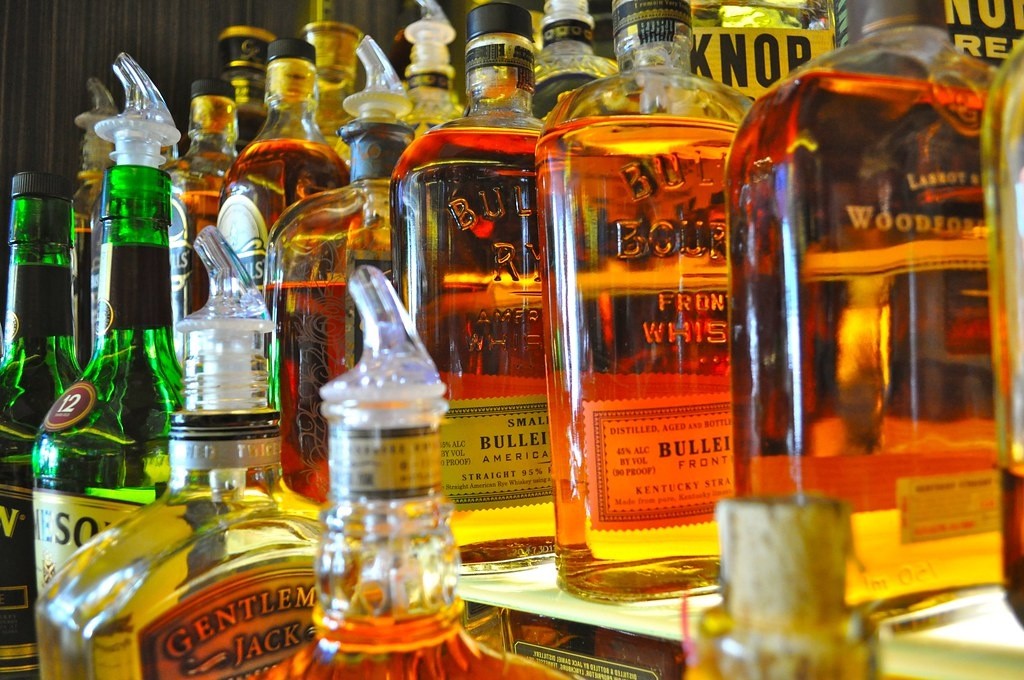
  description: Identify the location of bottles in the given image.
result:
[726,0,1006,638]
[220,36,352,234]
[300,21,363,163]
[70,75,121,368]
[336,33,416,265]
[34,225,327,679]
[170,77,240,315]
[402,0,464,122]
[90,51,190,361]
[1,169,85,680]
[33,165,186,680]
[250,264,574,679]
[532,0,620,119]
[534,1,756,606]
[979,37,1024,628]
[217,25,277,147]
[695,497,879,678]
[388,0,547,577]
